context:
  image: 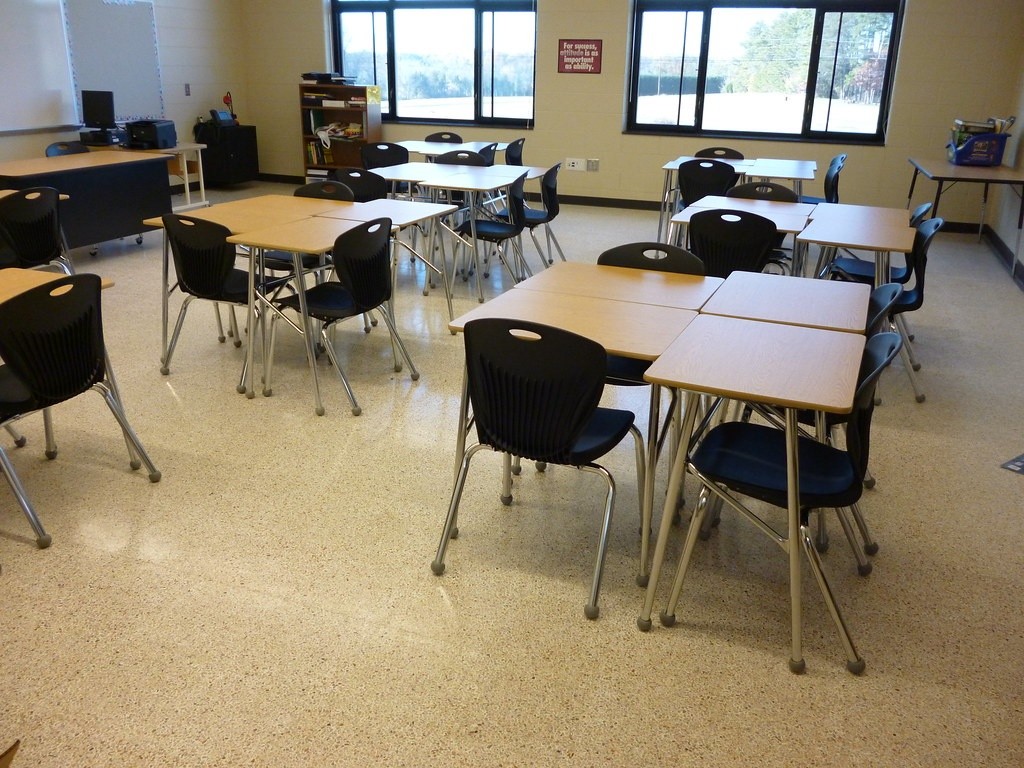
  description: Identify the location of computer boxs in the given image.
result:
[80,130,126,146]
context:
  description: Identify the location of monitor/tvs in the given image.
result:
[81,90,116,134]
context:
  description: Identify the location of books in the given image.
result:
[951,117,1007,161]
[301,72,357,84]
[307,141,325,165]
[303,92,366,105]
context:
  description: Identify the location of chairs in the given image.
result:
[159,151,559,412]
[425,132,463,161]
[360,142,418,199]
[0,273,160,548]
[505,138,526,165]
[44,141,89,157]
[475,142,498,165]
[431,245,945,674]
[673,184,942,404]
[658,147,848,234]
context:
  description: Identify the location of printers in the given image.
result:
[126,119,176,150]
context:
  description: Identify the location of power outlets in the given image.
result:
[565,159,598,173]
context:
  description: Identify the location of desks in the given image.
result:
[459,164,553,272]
[213,195,370,334]
[638,313,868,673]
[514,261,725,416]
[418,172,527,303]
[905,157,1024,277]
[0,190,76,274]
[809,202,910,227]
[797,220,917,289]
[668,206,810,277]
[753,158,817,195]
[85,141,209,212]
[193,123,258,186]
[0,152,178,251]
[0,269,140,478]
[675,156,755,166]
[701,270,872,426]
[688,194,816,216]
[745,166,815,202]
[142,205,319,366]
[379,140,511,164]
[226,217,402,415]
[447,289,697,587]
[315,199,460,349]
[655,161,754,260]
[350,168,457,263]
[384,163,485,267]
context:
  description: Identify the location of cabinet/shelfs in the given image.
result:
[298,83,381,177]
